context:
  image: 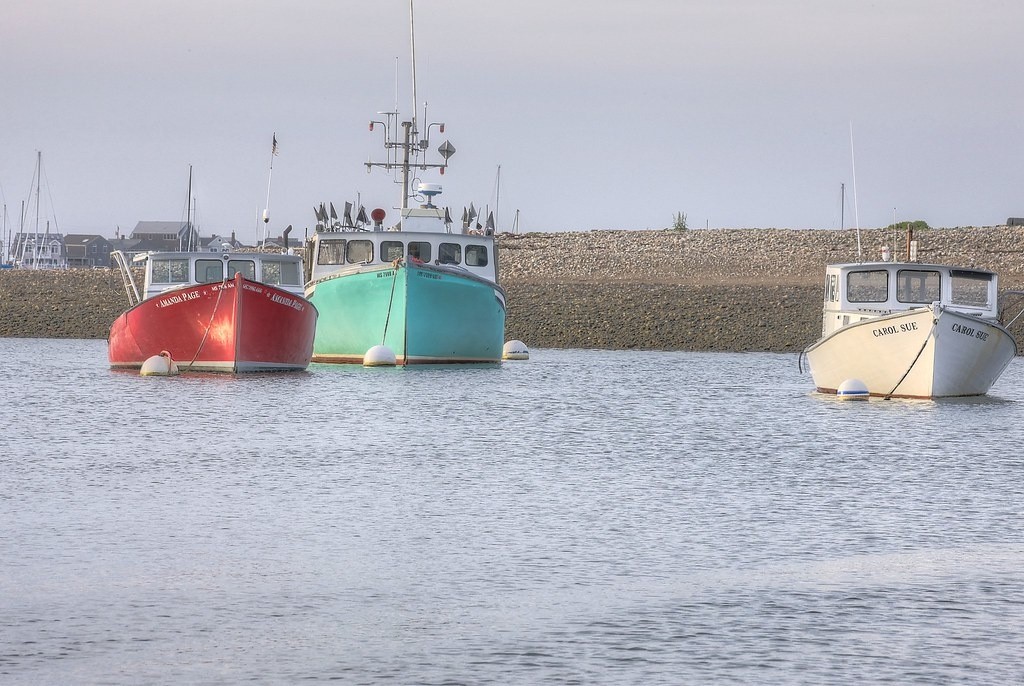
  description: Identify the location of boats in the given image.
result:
[107,131,320,373]
[305,0,532,367]
[797,119,1024,401]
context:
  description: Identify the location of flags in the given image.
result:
[444,205,495,231]
[314,202,369,228]
[272,134,280,158]
